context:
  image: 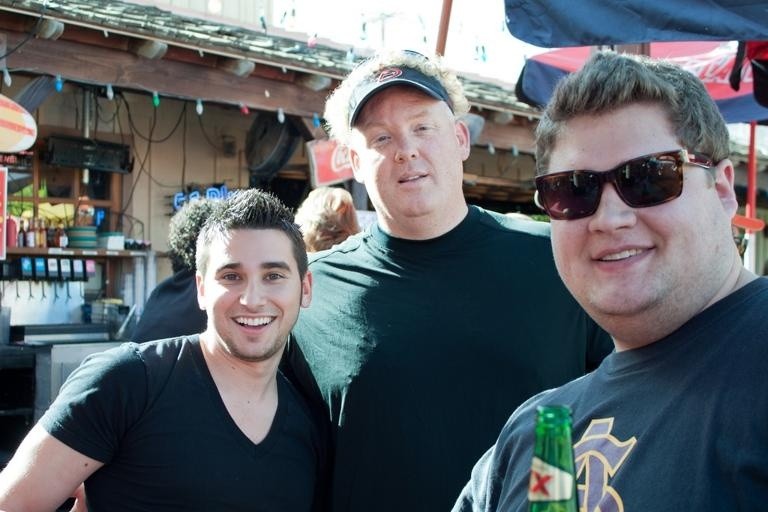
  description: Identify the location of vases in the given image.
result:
[64,226,98,248]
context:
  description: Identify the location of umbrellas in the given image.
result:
[504,0,768,44]
[515,41,767,237]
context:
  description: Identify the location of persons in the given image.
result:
[452,51,768,512]
[130,195,222,343]
[293,185,361,254]
[64,45,616,512]
[0,187,325,512]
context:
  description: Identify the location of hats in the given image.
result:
[347,50,456,131]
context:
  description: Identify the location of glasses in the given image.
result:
[533,148,716,221]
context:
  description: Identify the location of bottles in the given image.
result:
[526,402,580,512]
[16,217,68,248]
